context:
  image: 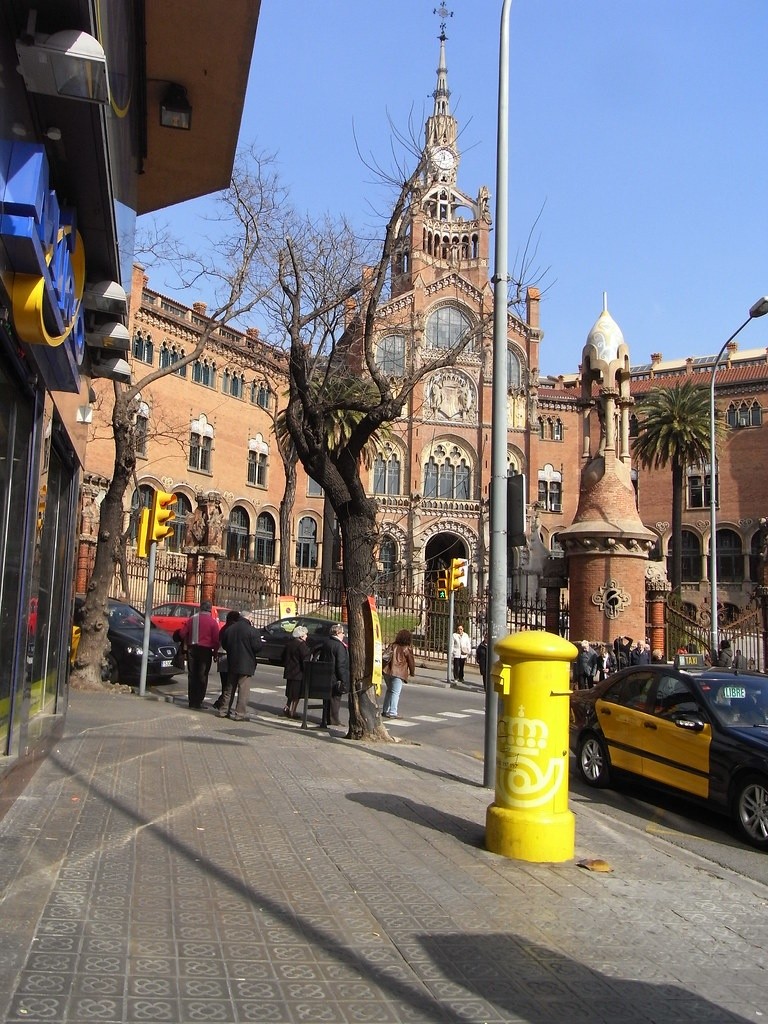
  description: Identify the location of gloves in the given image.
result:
[339,681,348,693]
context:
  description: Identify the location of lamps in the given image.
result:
[83,280,129,317]
[160,83,193,131]
[15,9,112,107]
[92,350,132,386]
[85,320,132,351]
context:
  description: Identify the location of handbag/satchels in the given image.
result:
[382,642,392,665]
[461,646,469,655]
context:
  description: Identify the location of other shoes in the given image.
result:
[212,701,231,714]
[189,702,208,709]
[455,677,465,682]
[288,711,301,719]
[383,712,403,719]
[283,706,290,715]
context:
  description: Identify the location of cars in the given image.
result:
[569,654,768,851]
[125,602,233,638]
[73,594,186,684]
[252,616,350,666]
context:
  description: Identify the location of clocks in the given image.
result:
[434,149,454,168]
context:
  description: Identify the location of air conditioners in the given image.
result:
[555,435,560,440]
[552,504,562,512]
[740,418,746,426]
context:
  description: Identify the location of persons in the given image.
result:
[381,629,416,719]
[595,644,618,681]
[734,650,747,670]
[179,601,220,711]
[319,623,348,728]
[452,623,472,683]
[476,630,488,692]
[677,645,688,654]
[281,626,312,719]
[573,639,600,690]
[629,640,667,667]
[211,610,260,722]
[613,635,633,672]
[712,640,733,668]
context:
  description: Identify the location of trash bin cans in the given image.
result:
[71,626,82,666]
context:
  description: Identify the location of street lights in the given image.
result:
[708,294,768,663]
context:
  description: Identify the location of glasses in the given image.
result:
[336,632,344,634]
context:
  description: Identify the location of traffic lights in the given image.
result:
[436,569,448,601]
[451,557,465,592]
[150,491,177,542]
